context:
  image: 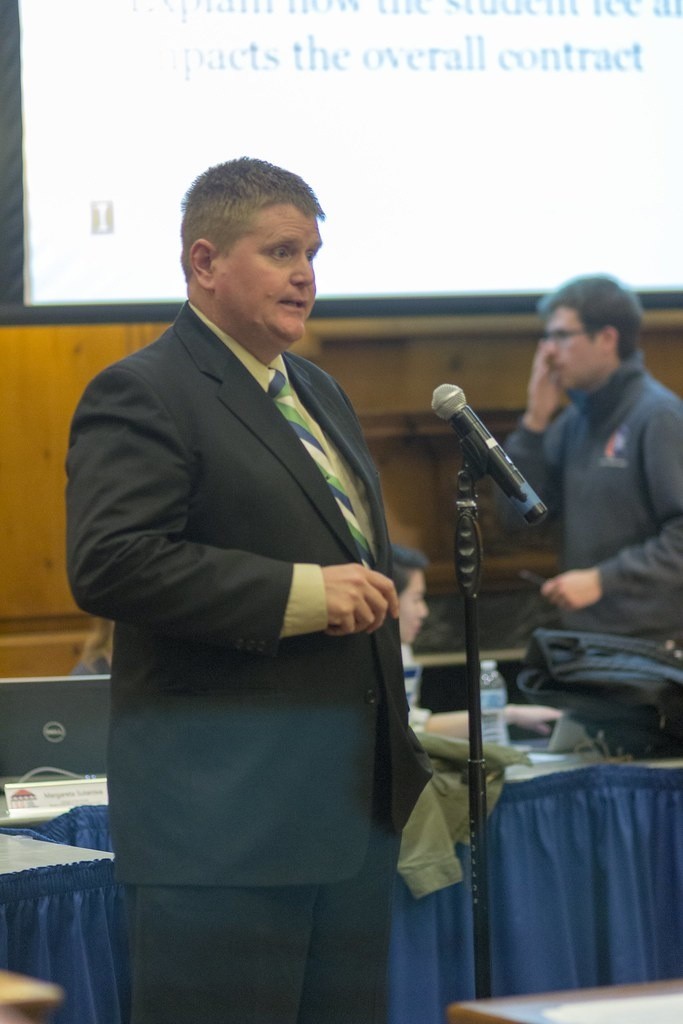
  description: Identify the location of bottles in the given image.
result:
[479,658,510,746]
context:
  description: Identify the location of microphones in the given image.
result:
[431,384,547,526]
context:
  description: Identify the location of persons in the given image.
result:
[390,543,562,739]
[65,157,432,1024]
[495,274,683,657]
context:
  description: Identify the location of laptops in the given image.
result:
[0,675,112,794]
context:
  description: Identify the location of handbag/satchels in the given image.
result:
[517,626,682,756]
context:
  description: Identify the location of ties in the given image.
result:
[263,367,373,573]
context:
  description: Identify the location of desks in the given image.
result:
[0,826,128,1024]
[0,737,683,1024]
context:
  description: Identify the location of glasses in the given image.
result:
[541,329,601,344]
